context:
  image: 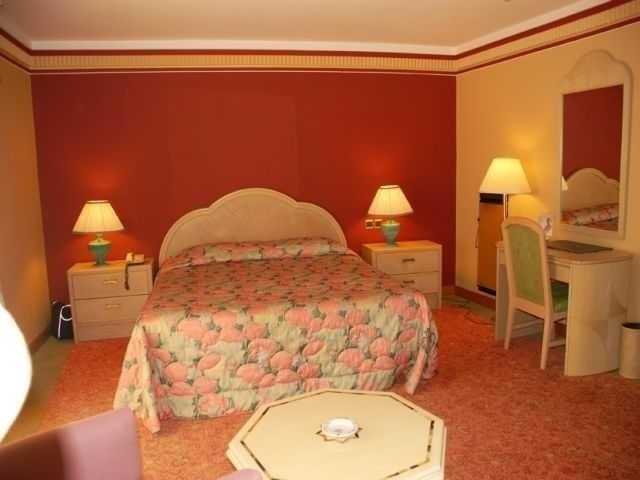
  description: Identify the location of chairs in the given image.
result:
[500,217,568,369]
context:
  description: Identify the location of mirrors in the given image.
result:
[552,49,633,240]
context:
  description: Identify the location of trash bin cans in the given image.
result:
[619,322,639,379]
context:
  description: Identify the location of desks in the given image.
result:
[226,388,446,479]
[495,239,632,376]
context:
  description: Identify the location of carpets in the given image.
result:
[37,301,640,480]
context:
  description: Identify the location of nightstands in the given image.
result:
[361,240,441,312]
[67,257,155,344]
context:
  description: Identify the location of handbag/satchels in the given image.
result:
[49,297,72,340]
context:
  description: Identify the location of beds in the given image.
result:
[560,166,619,233]
[112,187,439,434]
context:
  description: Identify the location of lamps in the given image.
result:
[479,158,531,220]
[368,184,413,247]
[72,198,124,267]
